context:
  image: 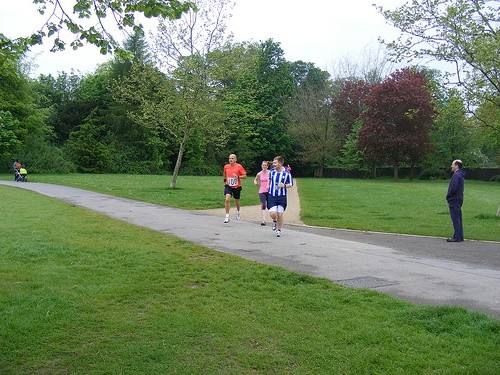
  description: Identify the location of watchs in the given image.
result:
[284,184,286,188]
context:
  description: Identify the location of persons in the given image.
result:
[18,161,22,171]
[446,159,466,242]
[13,159,19,180]
[223,154,247,223]
[254,161,270,226]
[266,156,293,237]
[286,165,291,170]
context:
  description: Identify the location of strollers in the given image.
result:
[14,168,27,182]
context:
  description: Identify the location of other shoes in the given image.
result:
[276,229,282,237]
[224,217,230,223]
[260,220,266,226]
[272,219,277,230]
[447,237,464,242]
[236,210,241,221]
